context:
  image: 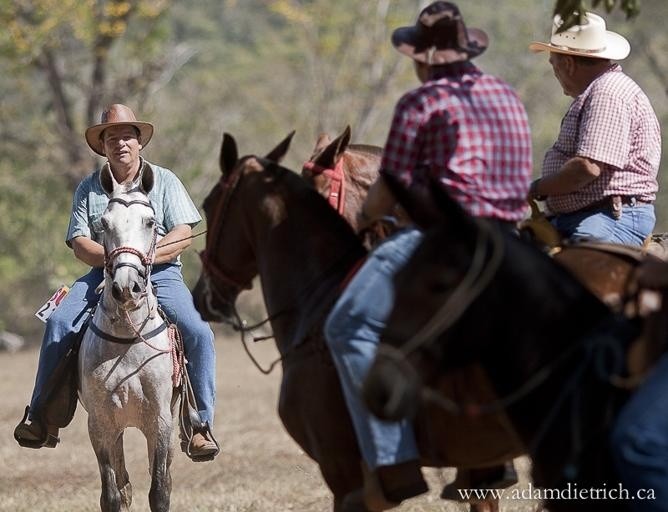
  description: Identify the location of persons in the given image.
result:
[531,11,662,248]
[14,104,222,464]
[323,0,534,512]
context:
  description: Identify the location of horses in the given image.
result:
[190,124,668,512]
[75,161,188,512]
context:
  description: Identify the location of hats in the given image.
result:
[85,104,154,157]
[529,11,631,61]
[391,1,489,65]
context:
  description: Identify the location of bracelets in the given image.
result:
[532,179,548,201]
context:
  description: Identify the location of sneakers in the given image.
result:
[180,432,219,457]
[340,460,430,512]
[15,418,59,449]
[440,464,519,501]
